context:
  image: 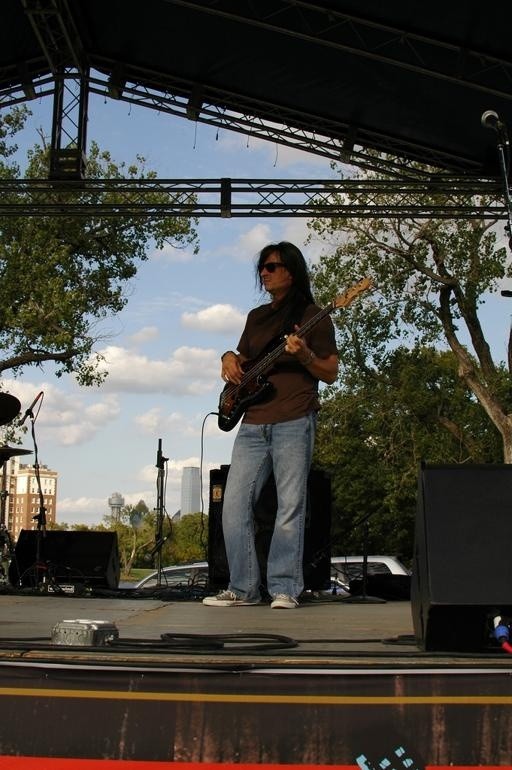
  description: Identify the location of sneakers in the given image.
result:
[270,593,298,609]
[202,589,260,606]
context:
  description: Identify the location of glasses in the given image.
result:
[257,262,288,272]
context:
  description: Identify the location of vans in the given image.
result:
[131,549,415,595]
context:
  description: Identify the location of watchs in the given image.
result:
[300,350,315,366]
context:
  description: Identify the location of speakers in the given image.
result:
[409,465,512,650]
[209,469,331,591]
[9,530,119,590]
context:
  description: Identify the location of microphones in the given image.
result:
[310,548,326,568]
[143,534,170,562]
[18,392,42,428]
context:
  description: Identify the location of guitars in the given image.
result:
[219,279,372,431]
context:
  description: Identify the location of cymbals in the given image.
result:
[0,445,33,457]
[0,392,20,426]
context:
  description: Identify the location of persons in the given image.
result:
[200,240,341,612]
